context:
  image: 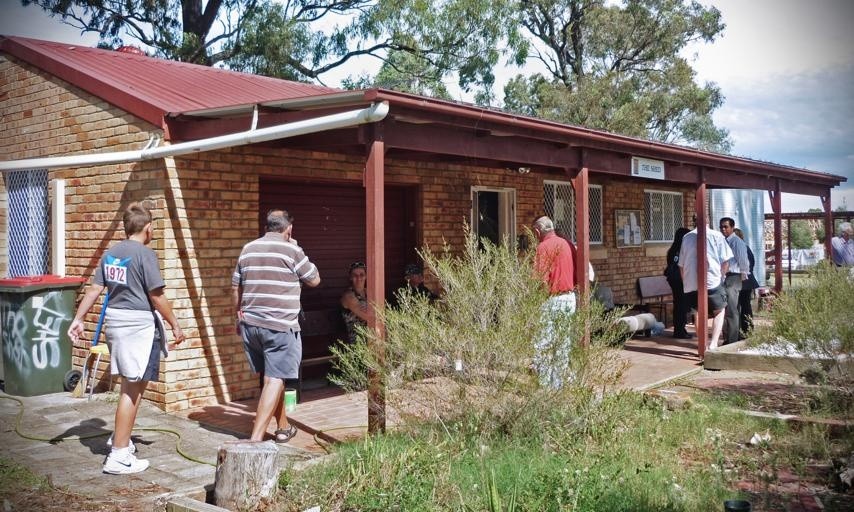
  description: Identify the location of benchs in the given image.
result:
[284,306,356,405]
[637,275,675,329]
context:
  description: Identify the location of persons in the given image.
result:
[824,222,854,284]
[68,205,183,476]
[528,217,577,392]
[340,261,367,346]
[663,210,758,350]
[395,264,444,314]
[230,210,321,443]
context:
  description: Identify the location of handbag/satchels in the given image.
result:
[664,256,682,288]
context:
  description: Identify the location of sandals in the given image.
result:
[274,423,297,443]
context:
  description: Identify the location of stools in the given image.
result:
[82,344,114,401]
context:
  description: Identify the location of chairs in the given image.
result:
[595,286,656,338]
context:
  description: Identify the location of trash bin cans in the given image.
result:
[0,275,88,397]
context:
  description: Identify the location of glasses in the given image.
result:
[720,225,729,228]
[350,262,365,269]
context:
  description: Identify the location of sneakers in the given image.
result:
[107,432,136,454]
[102,451,150,475]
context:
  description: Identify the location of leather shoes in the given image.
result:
[673,333,692,339]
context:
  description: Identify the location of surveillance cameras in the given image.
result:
[518,166,531,175]
